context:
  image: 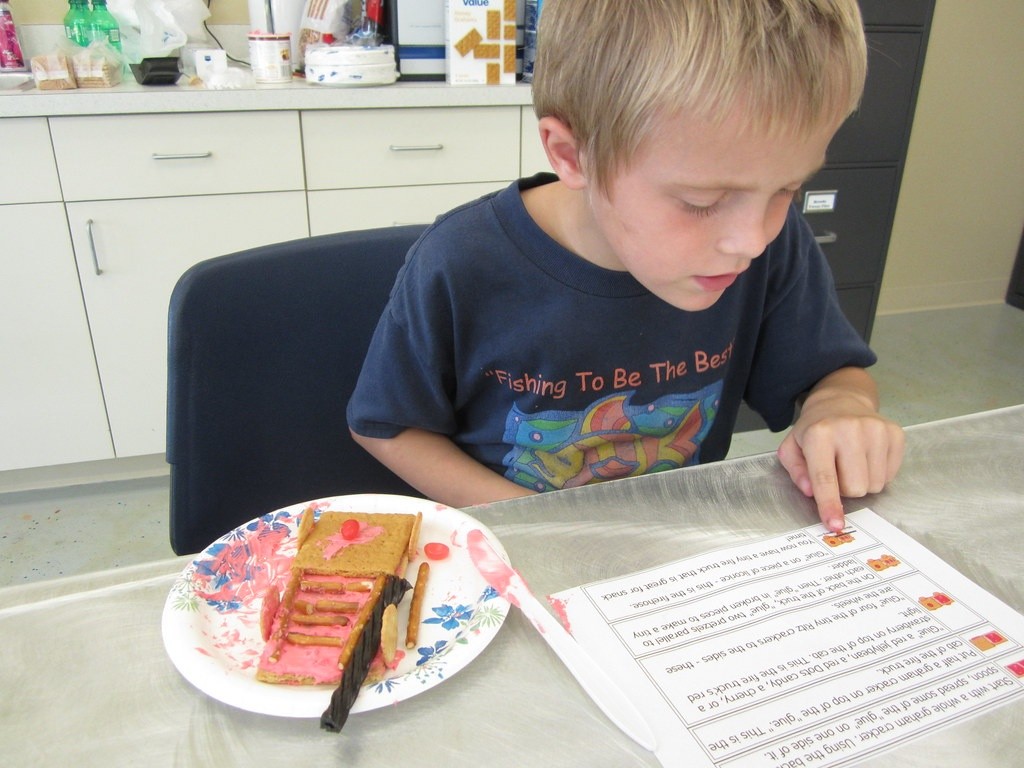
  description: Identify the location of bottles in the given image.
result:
[64,0,122,55]
[0,0,27,73]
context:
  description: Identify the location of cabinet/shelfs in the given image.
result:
[796,0,937,348]
[1,111,310,496]
[302,105,558,237]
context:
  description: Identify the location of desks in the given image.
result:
[0,404,1024,768]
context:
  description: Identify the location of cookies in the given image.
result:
[72,61,121,88]
[258,505,429,686]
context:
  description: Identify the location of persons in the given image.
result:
[345,0,905,531]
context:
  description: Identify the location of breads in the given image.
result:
[28,54,77,89]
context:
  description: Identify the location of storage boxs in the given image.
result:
[445,0,516,86]
[523,0,544,84]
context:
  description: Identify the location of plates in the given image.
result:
[161,493,512,718]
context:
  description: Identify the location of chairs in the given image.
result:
[165,225,433,556]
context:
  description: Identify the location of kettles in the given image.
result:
[248,0,305,70]
